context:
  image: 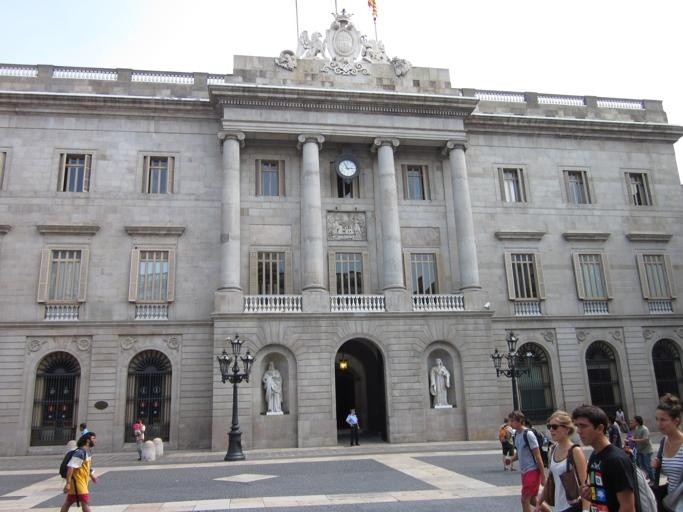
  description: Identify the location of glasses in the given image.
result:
[546,423,565,431]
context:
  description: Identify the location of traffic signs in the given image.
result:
[490,330,535,411]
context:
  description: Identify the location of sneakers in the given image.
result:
[350,443,361,446]
[504,467,518,472]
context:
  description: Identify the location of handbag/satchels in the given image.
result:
[651,481,670,512]
[623,446,635,458]
[559,444,582,511]
[543,468,555,506]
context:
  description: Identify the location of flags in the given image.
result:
[367,0,377,16]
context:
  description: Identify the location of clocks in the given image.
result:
[338,159,357,178]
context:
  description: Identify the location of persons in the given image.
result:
[135,417,146,460]
[496,390,682,510]
[79,422,95,475]
[430,358,450,406]
[133,420,141,440]
[345,408,361,446]
[262,360,284,412]
[60,433,98,511]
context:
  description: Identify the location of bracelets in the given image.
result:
[67,482,70,484]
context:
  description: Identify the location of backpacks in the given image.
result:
[60,448,87,479]
[499,425,512,445]
[631,459,660,512]
[515,427,553,467]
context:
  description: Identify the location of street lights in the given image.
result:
[216,331,256,461]
